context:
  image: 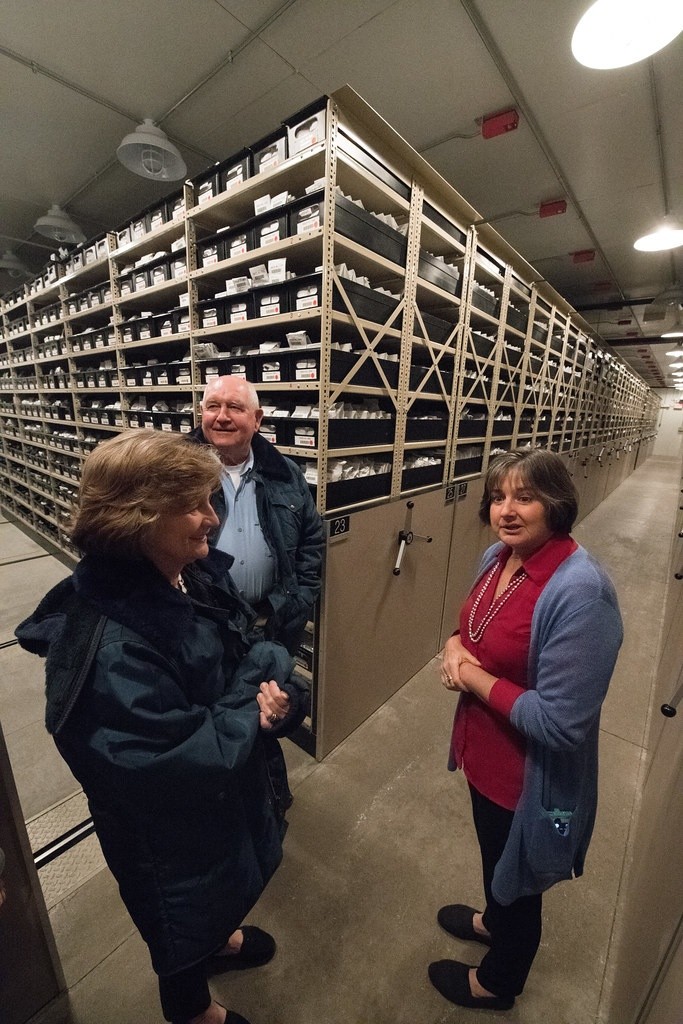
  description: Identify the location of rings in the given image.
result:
[448,677,453,680]
[271,714,277,721]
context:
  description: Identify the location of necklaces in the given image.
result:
[468,563,527,643]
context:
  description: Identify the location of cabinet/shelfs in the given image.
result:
[0,85,659,763]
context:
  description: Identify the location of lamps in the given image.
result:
[633,207,683,252]
[653,248,683,306]
[116,119,186,181]
[33,204,87,244]
[661,310,683,390]
[0,250,30,279]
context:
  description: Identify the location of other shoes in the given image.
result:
[173,999,251,1024]
[427,958,515,1011]
[213,925,278,973]
[438,904,491,946]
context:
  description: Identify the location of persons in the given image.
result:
[427,451,625,1011]
[14,427,312,1024]
[183,375,324,646]
